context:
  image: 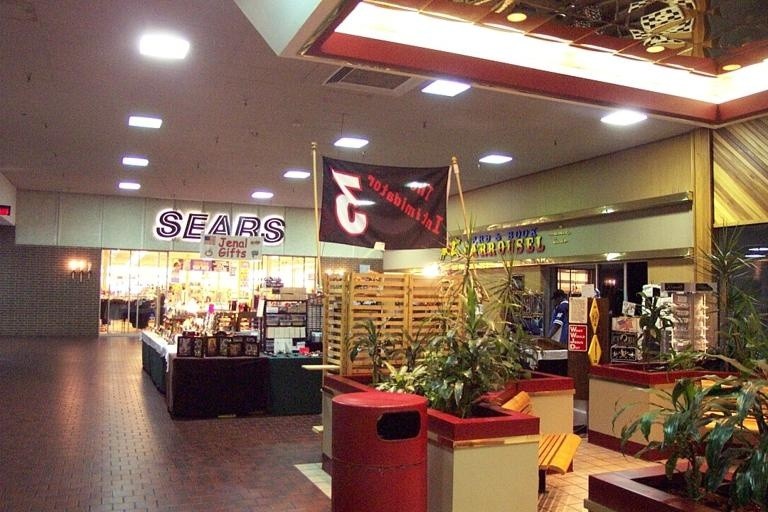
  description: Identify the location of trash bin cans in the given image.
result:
[331,392,430,511]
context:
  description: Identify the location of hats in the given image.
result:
[551,290,564,300]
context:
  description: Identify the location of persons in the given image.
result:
[548,289,569,345]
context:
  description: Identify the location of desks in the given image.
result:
[166,352,325,420]
[141,330,176,395]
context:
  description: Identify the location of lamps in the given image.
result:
[66,257,92,283]
[505,6,536,22]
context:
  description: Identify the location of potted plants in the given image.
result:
[589,295,768,461]
[585,221,768,512]
[321,211,574,512]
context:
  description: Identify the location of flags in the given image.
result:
[318,155,452,248]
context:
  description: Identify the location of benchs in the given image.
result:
[502,392,582,495]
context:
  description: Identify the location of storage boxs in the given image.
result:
[176,331,260,357]
